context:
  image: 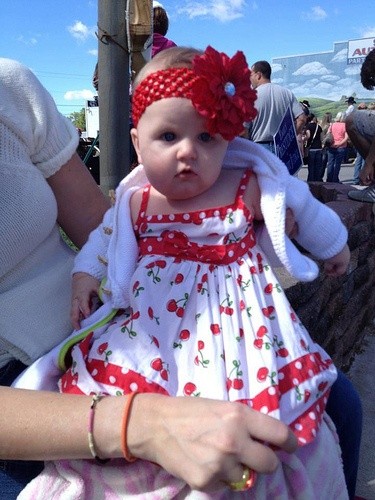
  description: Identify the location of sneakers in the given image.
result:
[348,184,375,202]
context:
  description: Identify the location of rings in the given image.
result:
[225,467,250,491]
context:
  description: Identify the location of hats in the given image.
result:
[346,97,356,103]
[358,103,366,108]
[299,101,310,108]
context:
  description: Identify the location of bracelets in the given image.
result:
[122,391,141,461]
[87,392,114,462]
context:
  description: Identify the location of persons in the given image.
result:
[71,45,353,453]
[353,102,367,185]
[242,60,306,160]
[299,100,311,115]
[152,5,177,60]
[347,47,375,204]
[322,113,333,182]
[0,58,361,500]
[344,97,356,117]
[327,112,349,183]
[304,113,323,181]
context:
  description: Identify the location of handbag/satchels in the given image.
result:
[324,124,334,146]
[303,148,309,165]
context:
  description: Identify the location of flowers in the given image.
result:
[191,45,259,143]
